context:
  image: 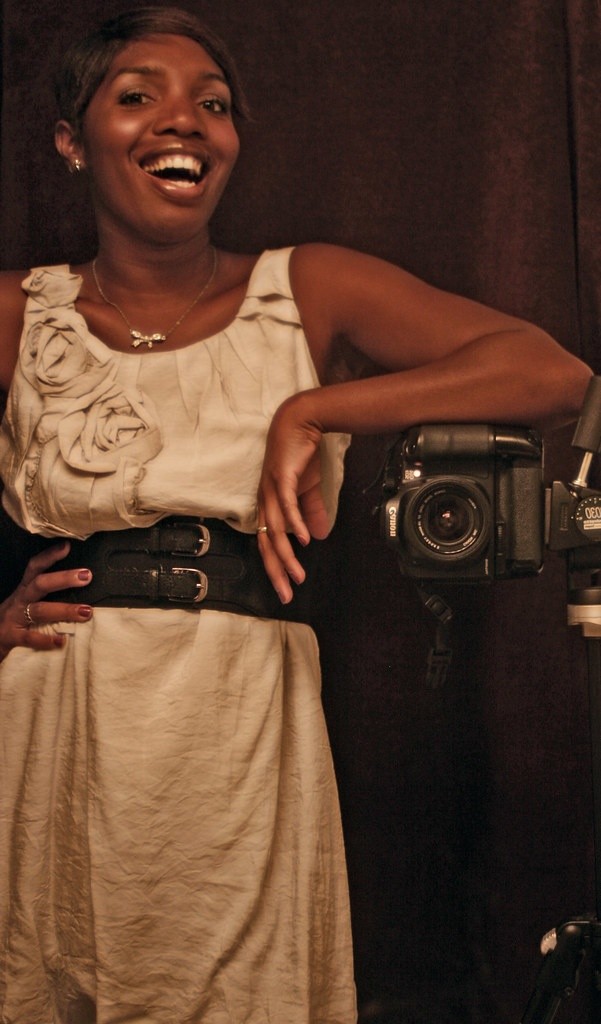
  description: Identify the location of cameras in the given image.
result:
[371,425,548,589]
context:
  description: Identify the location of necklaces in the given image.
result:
[93,242,218,347]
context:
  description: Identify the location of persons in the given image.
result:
[0,7,596,1024]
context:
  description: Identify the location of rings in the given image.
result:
[258,527,267,534]
[25,603,35,624]
